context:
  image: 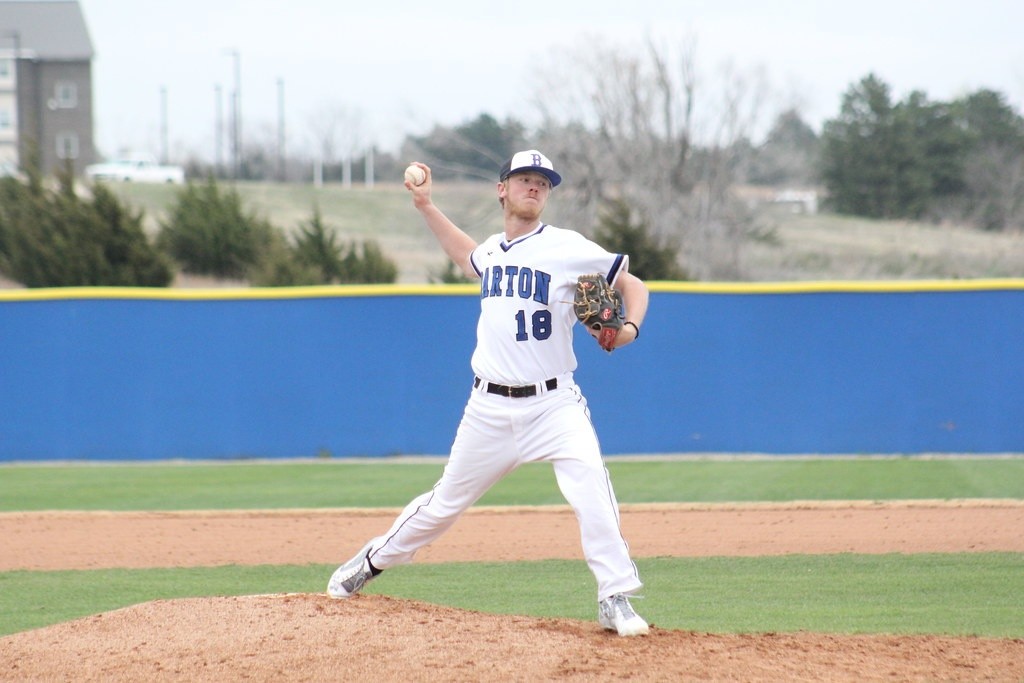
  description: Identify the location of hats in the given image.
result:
[499,150,561,188]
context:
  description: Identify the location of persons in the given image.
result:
[327,150,650,636]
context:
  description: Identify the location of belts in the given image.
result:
[474,377,557,397]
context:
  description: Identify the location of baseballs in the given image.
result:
[405,164,426,186]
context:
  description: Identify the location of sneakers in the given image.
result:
[327,537,385,597]
[599,592,648,637]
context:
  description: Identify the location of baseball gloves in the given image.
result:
[558,270,626,353]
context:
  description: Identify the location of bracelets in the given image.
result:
[624,321,639,338]
